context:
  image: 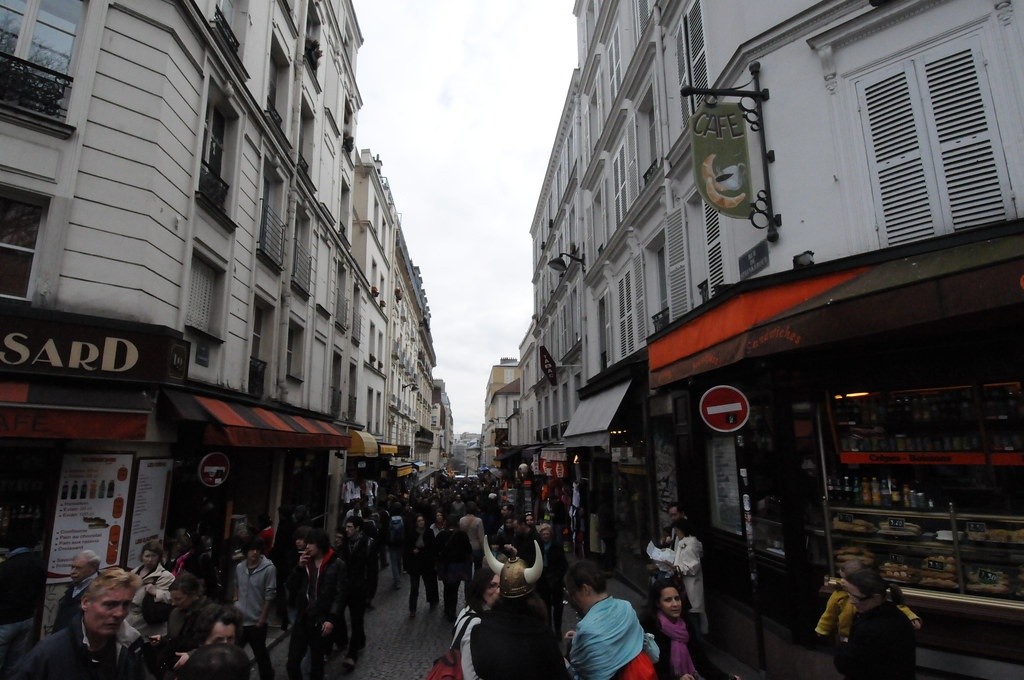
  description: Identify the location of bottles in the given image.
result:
[62,481,69,499]
[830,390,1024,452]
[107,480,115,498]
[80,481,87,498]
[0,504,42,536]
[71,480,78,499]
[89,480,97,498]
[98,480,105,498]
[826,476,933,509]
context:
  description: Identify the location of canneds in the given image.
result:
[908,490,928,508]
[839,433,1024,451]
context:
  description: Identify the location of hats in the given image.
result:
[394,502,402,510]
[488,493,497,499]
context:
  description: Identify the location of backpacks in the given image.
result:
[385,511,405,543]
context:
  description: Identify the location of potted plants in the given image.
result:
[0,12,70,118]
[215,182,227,203]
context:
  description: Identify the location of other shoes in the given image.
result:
[410,609,415,617]
[381,562,390,570]
[341,652,357,669]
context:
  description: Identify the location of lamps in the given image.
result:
[402,383,419,392]
[548,252,585,272]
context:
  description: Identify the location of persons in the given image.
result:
[815,559,922,680]
[0,478,703,680]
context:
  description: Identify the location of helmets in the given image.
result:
[483,534,543,598]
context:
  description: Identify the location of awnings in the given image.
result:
[493,445,566,460]
[158,390,353,452]
[380,443,426,477]
[562,378,633,448]
[348,429,379,457]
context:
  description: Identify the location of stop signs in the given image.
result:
[200,451,231,490]
[699,384,750,433]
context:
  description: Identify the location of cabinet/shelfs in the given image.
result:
[816,381,1024,624]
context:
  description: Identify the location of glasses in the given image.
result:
[488,581,500,588]
[524,511,532,516]
[563,587,571,597]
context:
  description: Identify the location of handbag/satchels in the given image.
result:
[643,632,660,663]
[141,576,176,624]
[432,561,445,573]
[127,634,175,680]
[426,608,478,680]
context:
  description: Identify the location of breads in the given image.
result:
[833,516,1024,597]
